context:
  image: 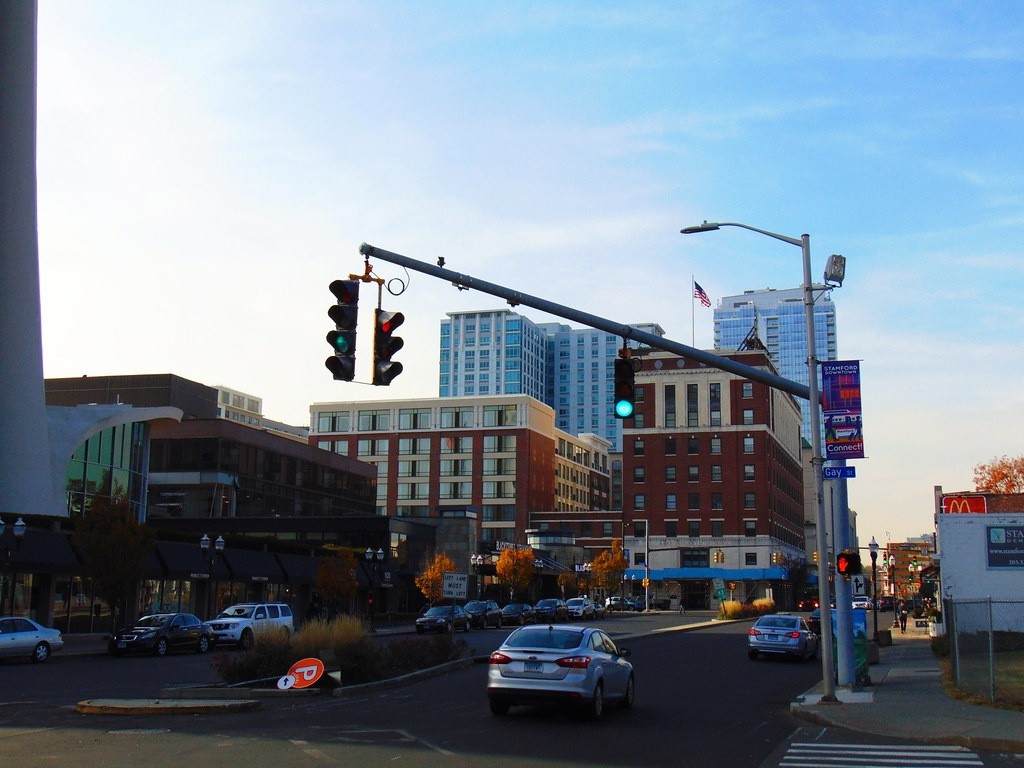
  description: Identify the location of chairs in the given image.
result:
[766,619,777,626]
[787,621,795,628]
[564,639,580,649]
[524,636,548,647]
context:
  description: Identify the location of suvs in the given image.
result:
[203,602,293,649]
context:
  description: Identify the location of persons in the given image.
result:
[898,601,910,633]
[594,595,605,607]
[680,599,686,614]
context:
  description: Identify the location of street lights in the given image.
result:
[680,220,840,704]
[869,536,879,641]
[909,564,924,610]
[199,533,225,621]
[0,517,28,616]
[471,554,483,600]
[889,555,899,628]
[365,547,385,632]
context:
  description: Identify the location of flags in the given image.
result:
[694,282,711,307]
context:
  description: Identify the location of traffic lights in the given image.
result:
[372,309,404,386]
[614,359,635,419]
[325,280,359,381]
[837,553,860,575]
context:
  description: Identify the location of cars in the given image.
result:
[797,594,920,612]
[0,616,63,662]
[748,614,818,662]
[415,599,537,634]
[485,624,631,718]
[108,613,216,657]
[533,596,644,621]
